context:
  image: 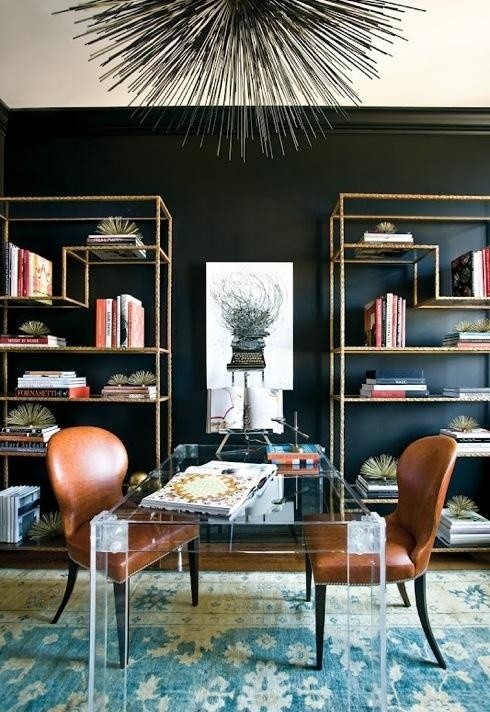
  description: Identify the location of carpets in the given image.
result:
[0,568,489,712]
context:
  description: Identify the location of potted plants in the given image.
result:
[211,270,289,352]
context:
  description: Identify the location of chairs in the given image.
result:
[47,424,201,670]
[305,435,458,670]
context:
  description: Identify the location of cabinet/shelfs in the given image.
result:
[2,195,173,550]
[328,191,490,554]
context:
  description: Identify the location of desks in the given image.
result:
[87,445,389,712]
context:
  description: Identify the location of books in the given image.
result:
[451,246,490,297]
[16,370,156,399]
[87,235,147,259]
[139,460,278,517]
[0,485,40,544]
[0,334,66,347]
[6,242,53,306]
[355,474,399,498]
[359,368,490,398]
[439,427,490,452]
[95,294,146,348]
[442,332,490,347]
[0,425,61,452]
[436,508,490,546]
[354,230,413,258]
[364,292,407,347]
